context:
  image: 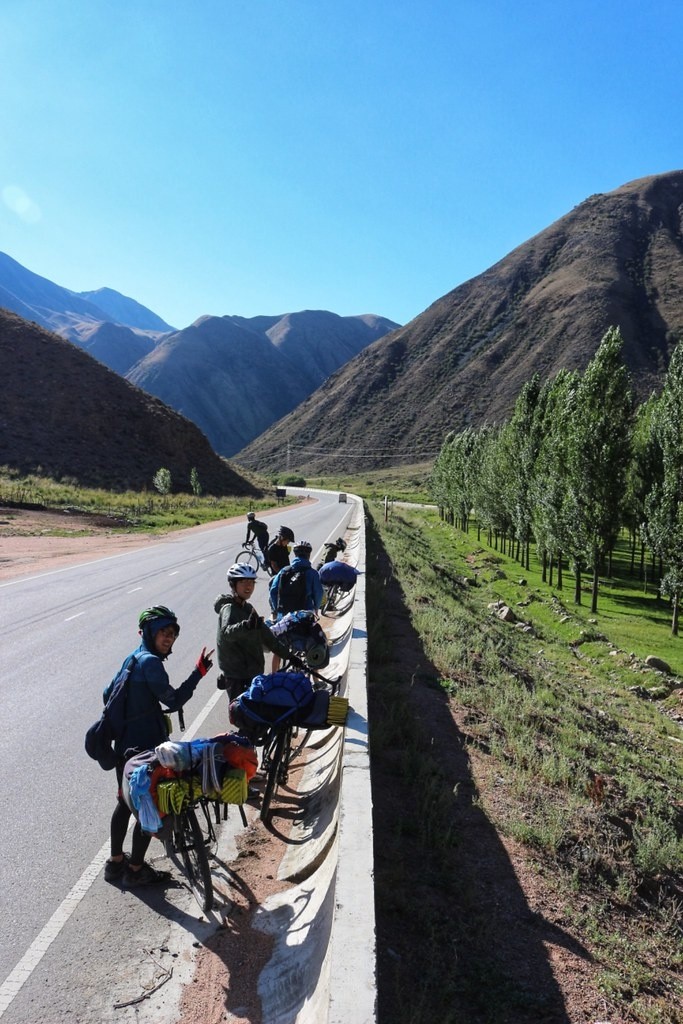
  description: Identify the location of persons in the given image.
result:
[242,512,348,692]
[213,562,303,799]
[102,607,216,888]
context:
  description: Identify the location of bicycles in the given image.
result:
[319,584,338,615]
[258,652,343,820]
[94,708,215,912]
[235,541,284,577]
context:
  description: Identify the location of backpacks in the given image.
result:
[257,522,267,531]
[276,565,310,613]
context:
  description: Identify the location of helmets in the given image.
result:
[247,512,255,517]
[278,525,296,543]
[293,540,313,548]
[227,563,257,578]
[138,605,176,629]
[336,537,347,548]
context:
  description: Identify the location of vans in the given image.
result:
[339,494,346,503]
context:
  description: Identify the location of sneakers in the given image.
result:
[104,851,132,881]
[122,861,172,887]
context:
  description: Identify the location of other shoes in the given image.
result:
[312,681,327,691]
[248,787,260,798]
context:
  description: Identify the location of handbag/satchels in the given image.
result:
[99,678,130,740]
